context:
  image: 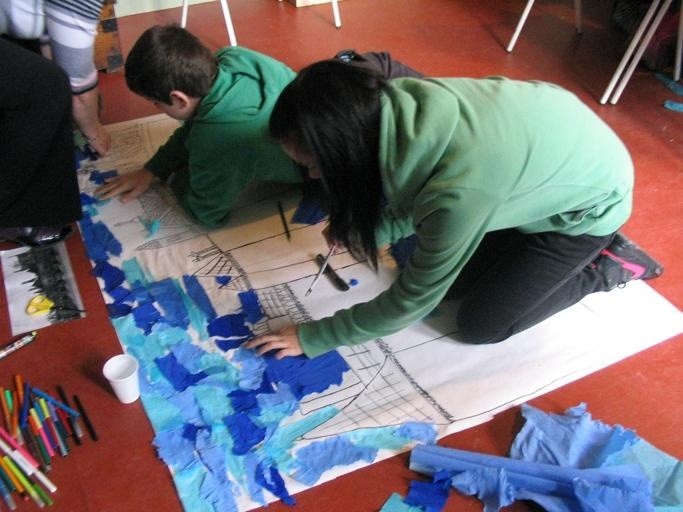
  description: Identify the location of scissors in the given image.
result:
[26,295,87,316]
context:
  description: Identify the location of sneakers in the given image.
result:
[592,233,664,292]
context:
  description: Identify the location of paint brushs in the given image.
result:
[276,201,290,241]
[304,243,338,296]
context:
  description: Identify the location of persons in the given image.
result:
[93,21,427,228]
[0,0,110,251]
[243,59,664,360]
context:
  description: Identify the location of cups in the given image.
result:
[102,353,141,404]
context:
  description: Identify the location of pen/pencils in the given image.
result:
[0,374,96,509]
[0,331,38,360]
[139,193,172,220]
[316,254,347,291]
